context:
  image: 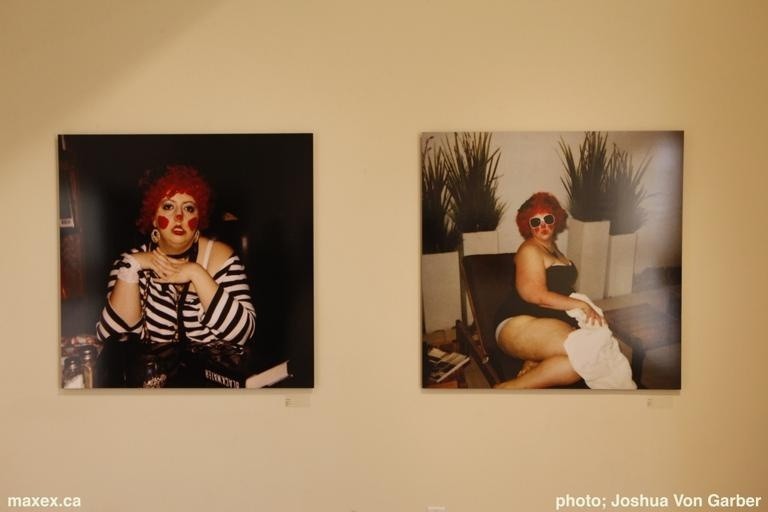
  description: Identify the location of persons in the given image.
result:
[94,163,257,388]
[492,192,608,390]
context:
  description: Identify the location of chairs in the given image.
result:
[456,252,594,390]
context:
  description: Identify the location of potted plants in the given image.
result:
[555,131,653,301]
[420,133,510,332]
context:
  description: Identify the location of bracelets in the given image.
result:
[116,254,141,284]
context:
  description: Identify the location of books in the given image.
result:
[202,351,290,389]
[427,347,470,383]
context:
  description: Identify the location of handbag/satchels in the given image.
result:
[98,335,250,387]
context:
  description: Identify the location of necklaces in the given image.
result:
[542,245,558,258]
[167,246,192,260]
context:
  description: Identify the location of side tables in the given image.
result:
[601,302,679,391]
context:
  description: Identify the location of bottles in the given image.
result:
[77,345,98,388]
[63,357,85,389]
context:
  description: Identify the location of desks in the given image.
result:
[62,341,313,386]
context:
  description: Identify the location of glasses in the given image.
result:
[529,214,556,229]
